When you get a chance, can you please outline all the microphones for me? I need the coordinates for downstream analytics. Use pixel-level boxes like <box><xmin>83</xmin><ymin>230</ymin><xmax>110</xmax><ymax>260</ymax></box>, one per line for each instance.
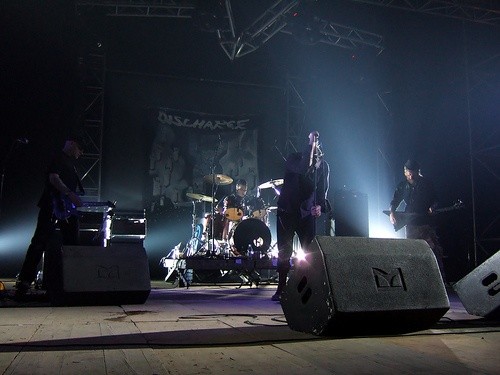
<box><xmin>316</xmin><ymin>145</ymin><xmax>324</xmax><ymax>155</ymax></box>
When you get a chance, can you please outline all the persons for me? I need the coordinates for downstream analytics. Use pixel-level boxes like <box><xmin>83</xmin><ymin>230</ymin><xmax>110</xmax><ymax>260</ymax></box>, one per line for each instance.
<box><xmin>272</xmin><ymin>132</ymin><xmax>330</xmax><ymax>300</ymax></box>
<box><xmin>17</xmin><ymin>135</ymin><xmax>88</xmax><ymax>293</ymax></box>
<box><xmin>388</xmin><ymin>160</ymin><xmax>446</xmax><ymax>285</ymax></box>
<box><xmin>215</xmin><ymin>179</ymin><xmax>254</xmax><ymax>241</ymax></box>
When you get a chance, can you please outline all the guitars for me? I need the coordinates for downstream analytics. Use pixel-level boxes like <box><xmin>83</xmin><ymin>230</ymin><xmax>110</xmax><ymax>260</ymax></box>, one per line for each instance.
<box><xmin>51</xmin><ymin>194</ymin><xmax>117</xmax><ymax>218</ymax></box>
<box><xmin>382</xmin><ymin>199</ymin><xmax>464</xmax><ymax>232</ymax></box>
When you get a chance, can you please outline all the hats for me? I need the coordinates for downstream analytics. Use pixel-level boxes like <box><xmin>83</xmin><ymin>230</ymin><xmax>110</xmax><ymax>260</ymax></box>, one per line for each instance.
<box><xmin>65</xmin><ymin>135</ymin><xmax>87</xmax><ymax>153</ymax></box>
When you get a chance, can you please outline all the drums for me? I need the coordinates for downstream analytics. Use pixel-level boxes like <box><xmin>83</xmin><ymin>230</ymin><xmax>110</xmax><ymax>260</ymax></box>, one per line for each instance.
<box><xmin>197</xmin><ymin>212</ymin><xmax>226</xmax><ymax>238</ymax></box>
<box><xmin>224</xmin><ymin>196</ymin><xmax>244</xmax><ymax>220</ymax></box>
<box><xmin>228</xmin><ymin>218</ymin><xmax>272</xmax><ymax>258</ymax></box>
<box><xmin>245</xmin><ymin>196</ymin><xmax>267</xmax><ymax>218</ymax></box>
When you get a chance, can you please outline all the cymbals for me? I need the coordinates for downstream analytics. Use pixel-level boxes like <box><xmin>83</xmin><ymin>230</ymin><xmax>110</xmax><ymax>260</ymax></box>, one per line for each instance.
<box><xmin>204</xmin><ymin>174</ymin><xmax>233</xmax><ymax>186</ymax></box>
<box><xmin>186</xmin><ymin>192</ymin><xmax>219</xmax><ymax>203</ymax></box>
<box><xmin>258</xmin><ymin>178</ymin><xmax>283</xmax><ymax>189</ymax></box>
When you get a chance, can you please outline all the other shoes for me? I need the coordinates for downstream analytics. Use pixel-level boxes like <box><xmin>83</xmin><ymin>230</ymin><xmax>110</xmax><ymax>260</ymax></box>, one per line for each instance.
<box><xmin>16</xmin><ymin>279</ymin><xmax>29</xmax><ymax>291</ymax></box>
<box><xmin>272</xmin><ymin>291</ymin><xmax>282</xmax><ymax>300</ymax></box>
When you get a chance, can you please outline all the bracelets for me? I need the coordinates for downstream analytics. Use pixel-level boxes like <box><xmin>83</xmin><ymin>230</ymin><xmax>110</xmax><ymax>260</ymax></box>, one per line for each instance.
<box><xmin>64</xmin><ymin>189</ymin><xmax>72</xmax><ymax>197</ymax></box>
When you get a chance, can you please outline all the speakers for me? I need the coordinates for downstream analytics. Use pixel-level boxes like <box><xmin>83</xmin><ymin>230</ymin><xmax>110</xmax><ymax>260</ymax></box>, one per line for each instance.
<box><xmin>279</xmin><ymin>235</ymin><xmax>451</xmax><ymax>338</ymax></box>
<box><xmin>452</xmin><ymin>250</ymin><xmax>500</xmax><ymax>327</ymax></box>
<box><xmin>45</xmin><ymin>213</ymin><xmax>152</xmax><ymax>306</ymax></box>
<box><xmin>315</xmin><ymin>192</ymin><xmax>369</xmax><ymax>237</ymax></box>
